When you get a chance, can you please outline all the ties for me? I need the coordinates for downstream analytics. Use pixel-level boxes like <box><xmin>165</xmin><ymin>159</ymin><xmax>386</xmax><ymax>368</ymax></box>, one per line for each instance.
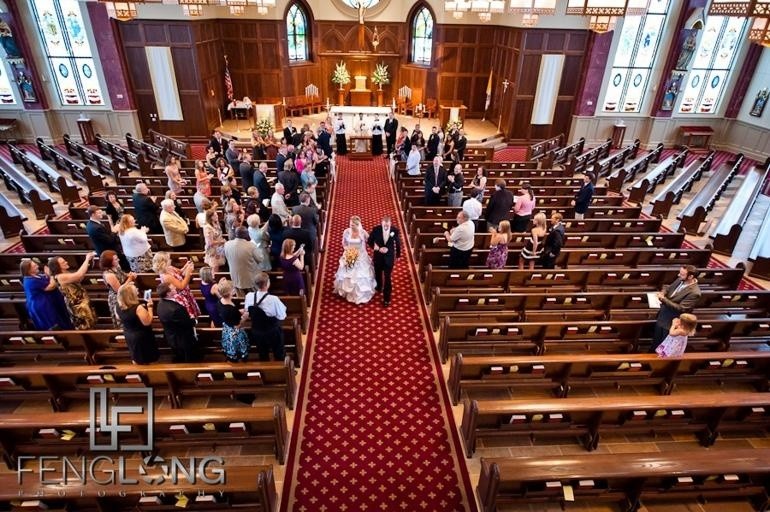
<box><xmin>435</xmin><ymin>168</ymin><xmax>438</xmax><ymax>186</ymax></box>
<box><xmin>384</xmin><ymin>231</ymin><xmax>388</xmax><ymax>245</ymax></box>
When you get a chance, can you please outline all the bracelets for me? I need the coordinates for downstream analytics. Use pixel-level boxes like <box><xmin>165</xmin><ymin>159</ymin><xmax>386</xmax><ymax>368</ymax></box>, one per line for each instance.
<box><xmin>377</xmin><ymin>247</ymin><xmax>381</xmax><ymax>252</ymax></box>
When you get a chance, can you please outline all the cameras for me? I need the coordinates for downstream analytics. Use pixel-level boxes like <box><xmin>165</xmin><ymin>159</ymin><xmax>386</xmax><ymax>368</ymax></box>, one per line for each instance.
<box><xmin>92</xmin><ymin>251</ymin><xmax>97</xmax><ymax>256</ymax></box>
<box><xmin>301</xmin><ymin>244</ymin><xmax>304</xmax><ymax>248</ymax></box>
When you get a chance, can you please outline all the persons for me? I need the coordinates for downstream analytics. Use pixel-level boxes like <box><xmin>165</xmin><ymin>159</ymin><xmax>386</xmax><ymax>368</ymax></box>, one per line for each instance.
<box><xmin>675</xmin><ymin>29</ymin><xmax>697</xmax><ymax>70</ymax></box>
<box><xmin>655</xmin><ymin>312</ymin><xmax>697</xmax><ymax>360</ymax></box>
<box><xmin>649</xmin><ymin>264</ymin><xmax>700</xmax><ymax>354</ymax></box>
<box><xmin>370</xmin><ymin>113</ymin><xmax>384</xmax><ymax>155</ymax></box>
<box><xmin>368</xmin><ymin>217</ymin><xmax>400</xmax><ymax>307</ymax></box>
<box><xmin>570</xmin><ymin>173</ymin><xmax>595</xmax><ymax>225</ymax></box>
<box><xmin>395</xmin><ymin>121</ymin><xmax>569</xmax><ymax>270</ymax></box>
<box><xmin>384</xmin><ymin>112</ymin><xmax>397</xmax><ymax>160</ymax></box>
<box><xmin>354</xmin><ymin>112</ymin><xmax>370</xmax><ymax>152</ymax></box>
<box><xmin>334</xmin><ymin>113</ymin><xmax>348</xmax><ymax>156</ymax></box>
<box><xmin>334</xmin><ymin>215</ymin><xmax>389</xmax><ymax>305</ymax></box>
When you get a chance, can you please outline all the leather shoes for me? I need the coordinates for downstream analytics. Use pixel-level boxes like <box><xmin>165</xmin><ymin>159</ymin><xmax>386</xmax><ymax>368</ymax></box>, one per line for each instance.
<box><xmin>384</xmin><ymin>300</ymin><xmax>389</xmax><ymax>307</ymax></box>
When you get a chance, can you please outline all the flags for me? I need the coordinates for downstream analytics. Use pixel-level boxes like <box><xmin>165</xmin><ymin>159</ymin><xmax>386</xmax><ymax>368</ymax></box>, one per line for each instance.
<box><xmin>224</xmin><ymin>61</ymin><xmax>234</xmax><ymax>102</ymax></box>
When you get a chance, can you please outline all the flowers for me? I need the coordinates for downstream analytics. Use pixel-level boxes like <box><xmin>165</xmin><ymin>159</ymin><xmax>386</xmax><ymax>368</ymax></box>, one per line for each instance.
<box><xmin>253</xmin><ymin>112</ymin><xmax>274</xmax><ymax>138</ymax></box>
<box><xmin>341</xmin><ymin>246</ymin><xmax>360</xmax><ymax>271</ymax></box>
<box><xmin>443</xmin><ymin>117</ymin><xmax>464</xmax><ymax>134</ymax></box>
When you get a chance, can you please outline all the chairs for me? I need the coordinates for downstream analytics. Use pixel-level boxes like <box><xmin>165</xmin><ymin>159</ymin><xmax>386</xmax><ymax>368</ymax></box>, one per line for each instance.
<box><xmin>391</xmin><ymin>95</ymin><xmax>463</xmax><ymax>122</ymax></box>
<box><xmin>257</xmin><ymin>94</ymin><xmax>324</xmax><ymax>116</ymax></box>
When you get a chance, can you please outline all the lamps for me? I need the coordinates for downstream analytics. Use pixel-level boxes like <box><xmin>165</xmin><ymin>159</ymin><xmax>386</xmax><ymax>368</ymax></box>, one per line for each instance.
<box><xmin>564</xmin><ymin>0</ymin><xmax>652</xmax><ymax>36</ymax></box>
<box><xmin>93</xmin><ymin>0</ymin><xmax>162</xmax><ymax>25</ymax></box>
<box><xmin>445</xmin><ymin>0</ymin><xmax>558</xmax><ymax>29</ymax></box>
<box><xmin>160</xmin><ymin>0</ymin><xmax>221</xmax><ymax>20</ymax></box>
<box><xmin>216</xmin><ymin>1</ymin><xmax>256</xmax><ymax>17</ymax></box>
<box><xmin>247</xmin><ymin>0</ymin><xmax>278</xmax><ymax>16</ymax></box>
<box><xmin>707</xmin><ymin>1</ymin><xmax>770</xmax><ymax>52</ymax></box>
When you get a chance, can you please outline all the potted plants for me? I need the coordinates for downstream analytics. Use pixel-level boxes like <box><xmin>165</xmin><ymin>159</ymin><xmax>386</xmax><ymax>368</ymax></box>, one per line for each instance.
<box><xmin>366</xmin><ymin>61</ymin><xmax>392</xmax><ymax>91</ymax></box>
<box><xmin>331</xmin><ymin>59</ymin><xmax>351</xmax><ymax>89</ymax></box>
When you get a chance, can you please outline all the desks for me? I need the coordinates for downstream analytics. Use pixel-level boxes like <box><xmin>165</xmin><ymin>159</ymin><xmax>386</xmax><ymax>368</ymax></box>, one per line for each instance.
<box><xmin>348</xmin><ymin>136</ymin><xmax>375</xmax><ymax>162</ymax></box>
<box><xmin>227</xmin><ymin>98</ymin><xmax>256</xmax><ymax>120</ymax></box>
<box><xmin>330</xmin><ymin>106</ymin><xmax>393</xmax><ymax>131</ymax></box>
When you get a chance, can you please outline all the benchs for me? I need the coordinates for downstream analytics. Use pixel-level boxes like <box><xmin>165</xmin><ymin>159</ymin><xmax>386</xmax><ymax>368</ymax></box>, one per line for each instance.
<box><xmin>390</xmin><ymin>132</ymin><xmax>769</xmax><ymax>508</ymax></box>
<box><xmin>0</xmin><ymin>125</ymin><xmax>341</xmax><ymax>511</ymax></box>
